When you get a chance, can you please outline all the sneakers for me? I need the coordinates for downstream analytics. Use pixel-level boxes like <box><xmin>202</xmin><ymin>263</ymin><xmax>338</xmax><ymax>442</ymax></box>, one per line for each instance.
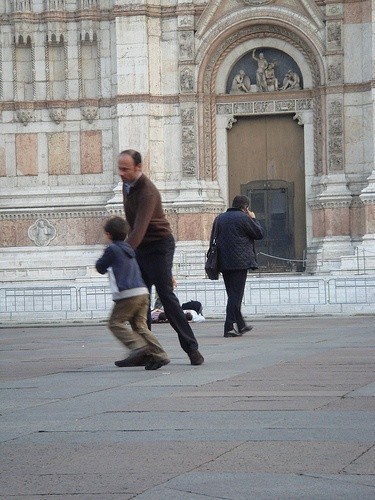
<box><xmin>115</xmin><ymin>358</ymin><xmax>146</xmax><ymax>366</ymax></box>
<box><xmin>188</xmin><ymin>350</ymin><xmax>204</xmax><ymax>364</ymax></box>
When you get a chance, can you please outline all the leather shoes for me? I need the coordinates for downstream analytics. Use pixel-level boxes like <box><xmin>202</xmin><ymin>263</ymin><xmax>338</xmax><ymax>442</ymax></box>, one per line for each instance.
<box><xmin>240</xmin><ymin>325</ymin><xmax>253</xmax><ymax>334</ymax></box>
<box><xmin>225</xmin><ymin>328</ymin><xmax>241</xmax><ymax>337</ymax></box>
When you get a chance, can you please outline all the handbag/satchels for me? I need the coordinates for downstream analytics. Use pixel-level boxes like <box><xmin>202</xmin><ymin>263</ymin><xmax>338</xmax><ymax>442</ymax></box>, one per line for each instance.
<box><xmin>205</xmin><ymin>243</ymin><xmax>219</xmax><ymax>280</ymax></box>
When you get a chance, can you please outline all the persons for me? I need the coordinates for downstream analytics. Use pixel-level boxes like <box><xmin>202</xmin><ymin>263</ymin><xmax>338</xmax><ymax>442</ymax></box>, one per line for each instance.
<box><xmin>209</xmin><ymin>194</ymin><xmax>265</xmax><ymax>337</ymax></box>
<box><xmin>118</xmin><ymin>149</ymin><xmax>205</xmax><ymax>365</ymax></box>
<box><xmin>155</xmin><ymin>278</ymin><xmax>177</xmax><ymax>310</ymax></box>
<box><xmin>231</xmin><ymin>48</ymin><xmax>300</xmax><ymax>93</ymax></box>
<box><xmin>95</xmin><ymin>217</ymin><xmax>171</xmax><ymax>371</ymax></box>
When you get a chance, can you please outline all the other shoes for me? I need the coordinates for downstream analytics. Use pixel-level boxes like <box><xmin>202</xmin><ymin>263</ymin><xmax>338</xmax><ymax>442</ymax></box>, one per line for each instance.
<box><xmin>127</xmin><ymin>346</ymin><xmax>154</xmax><ymax>363</ymax></box>
<box><xmin>144</xmin><ymin>358</ymin><xmax>170</xmax><ymax>370</ymax></box>
